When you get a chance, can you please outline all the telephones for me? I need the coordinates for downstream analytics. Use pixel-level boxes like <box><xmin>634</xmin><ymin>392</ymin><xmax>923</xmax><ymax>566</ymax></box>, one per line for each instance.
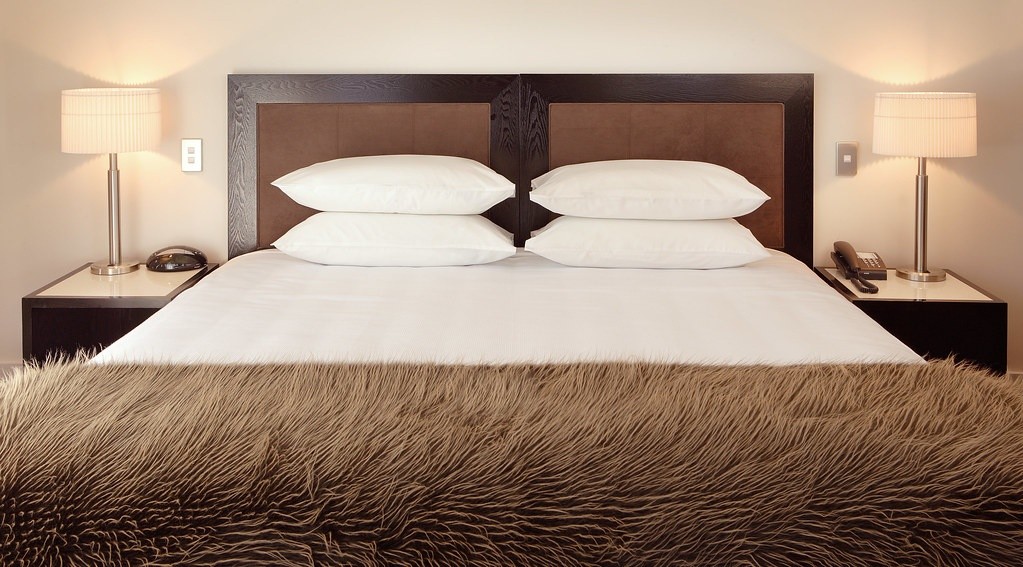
<box><xmin>831</xmin><ymin>240</ymin><xmax>887</xmax><ymax>280</ymax></box>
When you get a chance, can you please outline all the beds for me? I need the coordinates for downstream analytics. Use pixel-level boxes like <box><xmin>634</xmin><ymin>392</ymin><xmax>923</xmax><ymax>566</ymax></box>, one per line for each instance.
<box><xmin>2</xmin><ymin>72</ymin><xmax>1022</xmax><ymax>565</ymax></box>
